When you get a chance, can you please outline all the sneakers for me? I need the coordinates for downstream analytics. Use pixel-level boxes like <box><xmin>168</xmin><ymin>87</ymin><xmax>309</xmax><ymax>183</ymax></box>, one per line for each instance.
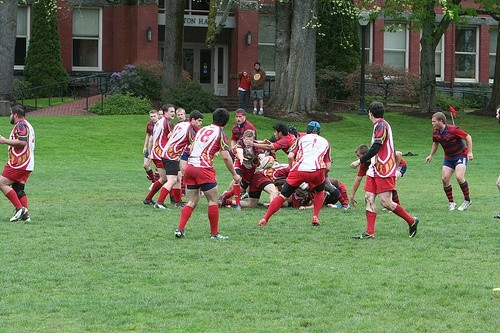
<box><xmin>10</xmin><ymin>206</ymin><xmax>28</xmax><ymax>221</ymax></box>
<box><xmin>21</xmin><ymin>216</ymin><xmax>30</xmax><ymax>222</ymax></box>
<box><xmin>409</xmin><ymin>217</ymin><xmax>419</xmax><ymax>238</ymax></box>
<box><xmin>458</xmin><ymin>200</ymin><xmax>472</xmax><ymax>211</ymax></box>
<box><xmin>449</xmin><ymin>203</ymin><xmax>456</xmax><ymax>211</ymax></box>
<box><xmin>352</xmin><ymin>232</ymin><xmax>375</xmax><ymax>239</ymax></box>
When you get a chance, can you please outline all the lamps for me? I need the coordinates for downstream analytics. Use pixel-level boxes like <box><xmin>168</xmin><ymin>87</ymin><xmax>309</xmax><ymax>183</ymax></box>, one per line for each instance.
<box><xmin>246</xmin><ymin>31</ymin><xmax>252</xmax><ymax>45</ymax></box>
<box><xmin>147</xmin><ymin>26</ymin><xmax>152</xmax><ymax>42</ymax></box>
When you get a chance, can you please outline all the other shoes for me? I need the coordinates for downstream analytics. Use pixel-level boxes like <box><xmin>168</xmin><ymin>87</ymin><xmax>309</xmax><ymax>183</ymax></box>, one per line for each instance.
<box><xmin>258</xmin><ymin>219</ymin><xmax>267</xmax><ymax>226</ymax></box>
<box><xmin>253</xmin><ymin>111</ymin><xmax>258</xmax><ymax>114</ymax></box>
<box><xmin>143</xmin><ymin>201</ymin><xmax>155</xmax><ymax>204</ymax></box>
<box><xmin>342</xmin><ymin>204</ymin><xmax>349</xmax><ymax>210</ymax></box>
<box><xmin>209</xmin><ymin>233</ymin><xmax>225</xmax><ymax>240</ymax></box>
<box><xmin>174</xmin><ymin>202</ymin><xmax>187</xmax><ymax>207</ymax></box>
<box><xmin>258</xmin><ymin>110</ymin><xmax>263</xmax><ymax>114</ymax></box>
<box><xmin>312</xmin><ymin>216</ymin><xmax>321</xmax><ymax>225</ymax></box>
<box><xmin>175</xmin><ymin>228</ymin><xmax>184</xmax><ymax>238</ymax></box>
<box><xmin>219</xmin><ymin>190</ymin><xmax>247</xmax><ymax>211</ymax></box>
<box><xmin>154</xmin><ymin>203</ymin><xmax>166</xmax><ymax>208</ymax></box>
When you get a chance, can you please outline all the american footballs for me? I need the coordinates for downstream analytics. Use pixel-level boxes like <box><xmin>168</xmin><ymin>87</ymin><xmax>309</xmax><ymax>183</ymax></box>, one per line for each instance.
<box><xmin>261</xmin><ymin>156</ymin><xmax>274</xmax><ymax>169</ymax></box>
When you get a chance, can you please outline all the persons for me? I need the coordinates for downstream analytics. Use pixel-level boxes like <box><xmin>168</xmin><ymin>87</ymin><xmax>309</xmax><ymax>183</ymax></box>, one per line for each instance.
<box><xmin>0</xmin><ymin>105</ymin><xmax>35</xmax><ymax>223</ymax></box>
<box><xmin>426</xmin><ymin>112</ymin><xmax>474</xmax><ymax>212</ymax></box>
<box><xmin>494</xmin><ymin>107</ymin><xmax>500</xmax><ymax>219</ymax></box>
<box><xmin>142</xmin><ymin>62</ymin><xmax>419</xmax><ymax>240</ymax></box>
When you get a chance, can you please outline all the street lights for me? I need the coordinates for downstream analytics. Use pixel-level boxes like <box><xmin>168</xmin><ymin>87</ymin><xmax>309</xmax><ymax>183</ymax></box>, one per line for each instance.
<box><xmin>356</xmin><ymin>7</ymin><xmax>372</xmax><ymax>115</ymax></box>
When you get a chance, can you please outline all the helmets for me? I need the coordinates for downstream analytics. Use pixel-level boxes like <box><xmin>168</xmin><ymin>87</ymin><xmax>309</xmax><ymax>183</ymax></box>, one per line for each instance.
<box><xmin>307</xmin><ymin>121</ymin><xmax>320</xmax><ymax>135</ymax></box>
